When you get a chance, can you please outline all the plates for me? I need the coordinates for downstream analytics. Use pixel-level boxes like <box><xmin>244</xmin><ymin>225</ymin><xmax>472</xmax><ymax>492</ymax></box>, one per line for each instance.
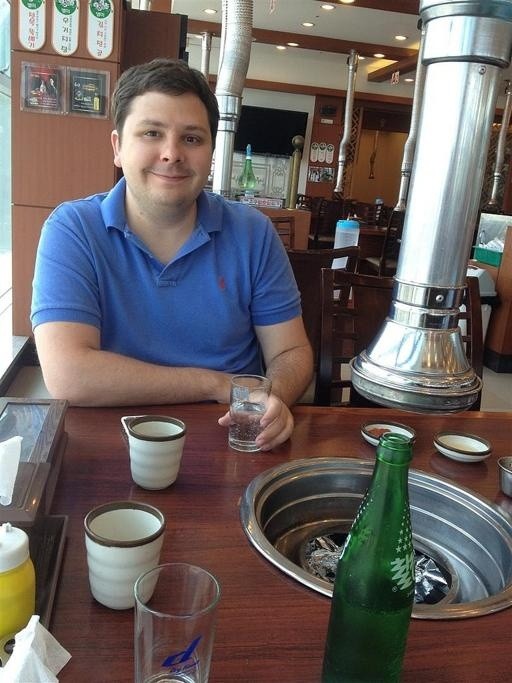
<box><xmin>361</xmin><ymin>420</ymin><xmax>417</xmax><ymax>449</ymax></box>
<box><xmin>432</xmin><ymin>429</ymin><xmax>492</xmax><ymax>463</ymax></box>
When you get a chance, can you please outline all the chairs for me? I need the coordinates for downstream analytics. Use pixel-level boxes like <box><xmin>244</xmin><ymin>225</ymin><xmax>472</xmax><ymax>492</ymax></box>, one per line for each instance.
<box><xmin>249</xmin><ymin>190</ymin><xmax>490</xmax><ymax>410</ymax></box>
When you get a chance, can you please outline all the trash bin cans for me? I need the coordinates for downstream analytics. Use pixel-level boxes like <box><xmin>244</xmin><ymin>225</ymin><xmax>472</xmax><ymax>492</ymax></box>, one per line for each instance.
<box><xmin>457</xmin><ymin>264</ymin><xmax>498</xmax><ymax>355</ymax></box>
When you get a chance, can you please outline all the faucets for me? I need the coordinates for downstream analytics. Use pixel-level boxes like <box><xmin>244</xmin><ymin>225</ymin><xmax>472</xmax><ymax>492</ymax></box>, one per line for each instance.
<box><xmin>477</xmin><ymin>228</ymin><xmax>486</xmax><ymax>243</ymax></box>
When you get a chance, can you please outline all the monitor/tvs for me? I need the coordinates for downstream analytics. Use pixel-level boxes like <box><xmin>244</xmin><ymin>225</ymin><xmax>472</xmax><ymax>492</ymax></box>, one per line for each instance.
<box><xmin>234</xmin><ymin>102</ymin><xmax>309</xmax><ymax>159</ymax></box>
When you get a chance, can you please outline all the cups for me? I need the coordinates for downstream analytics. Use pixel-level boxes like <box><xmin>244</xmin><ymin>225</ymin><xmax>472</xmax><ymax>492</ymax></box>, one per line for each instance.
<box><xmin>228</xmin><ymin>374</ymin><xmax>273</xmax><ymax>452</ymax></box>
<box><xmin>495</xmin><ymin>455</ymin><xmax>512</xmax><ymax>500</ymax></box>
<box><xmin>120</xmin><ymin>412</ymin><xmax>188</xmax><ymax>490</ymax></box>
<box><xmin>133</xmin><ymin>563</ymin><xmax>220</xmax><ymax>682</ymax></box>
<box><xmin>82</xmin><ymin>500</ymin><xmax>166</xmax><ymax>610</ymax></box>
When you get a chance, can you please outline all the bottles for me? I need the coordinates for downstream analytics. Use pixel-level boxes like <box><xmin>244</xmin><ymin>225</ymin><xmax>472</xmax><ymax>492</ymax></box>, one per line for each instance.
<box><xmin>322</xmin><ymin>433</ymin><xmax>418</xmax><ymax>682</ymax></box>
<box><xmin>238</xmin><ymin>142</ymin><xmax>256</xmax><ymax>189</ymax></box>
<box><xmin>0</xmin><ymin>519</ymin><xmax>38</xmax><ymax>670</ymax></box>
<box><xmin>329</xmin><ymin>220</ymin><xmax>361</xmax><ymax>299</ymax></box>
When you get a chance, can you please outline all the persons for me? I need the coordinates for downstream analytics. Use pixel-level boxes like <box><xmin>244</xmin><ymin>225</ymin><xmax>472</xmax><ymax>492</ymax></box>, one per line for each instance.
<box><xmin>30</xmin><ymin>58</ymin><xmax>315</xmax><ymax>452</ymax></box>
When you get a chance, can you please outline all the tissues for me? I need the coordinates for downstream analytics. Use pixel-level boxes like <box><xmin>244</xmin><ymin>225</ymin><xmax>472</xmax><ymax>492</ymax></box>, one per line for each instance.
<box><xmin>0</xmin><ymin>430</ymin><xmax>52</xmax><ymax>547</ymax></box>
<box><xmin>0</xmin><ymin>620</ymin><xmax>66</xmax><ymax>683</ymax></box>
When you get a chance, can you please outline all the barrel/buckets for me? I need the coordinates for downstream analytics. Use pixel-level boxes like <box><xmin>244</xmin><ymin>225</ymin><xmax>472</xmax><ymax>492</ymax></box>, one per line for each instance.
<box><xmin>330</xmin><ymin>215</ymin><xmax>361</xmax><ymax>271</ymax></box>
